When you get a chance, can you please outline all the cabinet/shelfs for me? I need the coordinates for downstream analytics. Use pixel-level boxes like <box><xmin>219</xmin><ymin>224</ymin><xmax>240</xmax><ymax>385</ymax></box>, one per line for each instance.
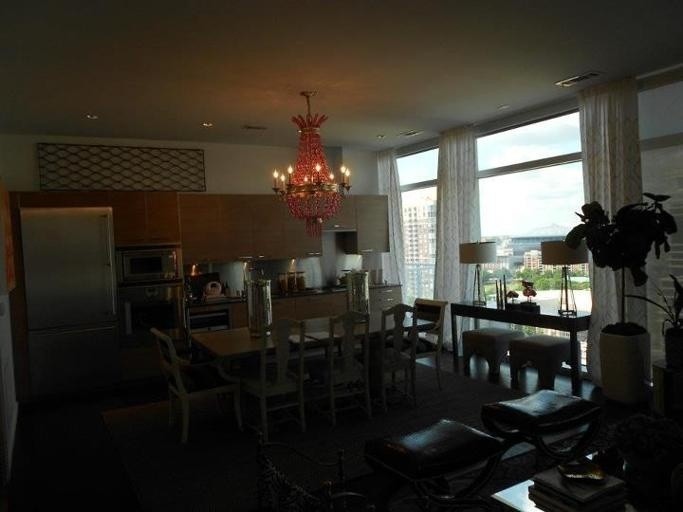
<box><xmin>110</xmin><ymin>191</ymin><xmax>182</xmax><ymax>248</ymax></box>
<box><xmin>176</xmin><ymin>194</ymin><xmax>393</xmax><ymax>262</ymax></box>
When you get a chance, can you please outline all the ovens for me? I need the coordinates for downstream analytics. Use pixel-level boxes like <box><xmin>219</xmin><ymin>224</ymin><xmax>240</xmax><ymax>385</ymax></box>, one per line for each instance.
<box><xmin>118</xmin><ymin>286</ymin><xmax>185</xmax><ymax>337</ymax></box>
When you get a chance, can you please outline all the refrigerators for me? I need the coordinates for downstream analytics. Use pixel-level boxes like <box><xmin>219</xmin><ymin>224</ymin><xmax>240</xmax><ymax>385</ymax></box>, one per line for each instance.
<box><xmin>17</xmin><ymin>206</ymin><xmax>117</xmax><ymax>414</ymax></box>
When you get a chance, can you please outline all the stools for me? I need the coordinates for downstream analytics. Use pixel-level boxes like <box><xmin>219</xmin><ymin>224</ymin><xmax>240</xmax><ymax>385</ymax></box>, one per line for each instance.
<box><xmin>370</xmin><ymin>415</ymin><xmax>510</xmax><ymax>511</ymax></box>
<box><xmin>460</xmin><ymin>326</ymin><xmax>523</xmax><ymax>384</ymax></box>
<box><xmin>477</xmin><ymin>383</ymin><xmax>601</xmax><ymax>472</ymax></box>
<box><xmin>508</xmin><ymin>335</ymin><xmax>581</xmax><ymax>391</ymax></box>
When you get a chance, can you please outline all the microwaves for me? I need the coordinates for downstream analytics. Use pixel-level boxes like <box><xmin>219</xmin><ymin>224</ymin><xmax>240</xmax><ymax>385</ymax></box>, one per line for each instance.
<box><xmin>122</xmin><ymin>248</ymin><xmax>177</xmax><ymax>278</ymax></box>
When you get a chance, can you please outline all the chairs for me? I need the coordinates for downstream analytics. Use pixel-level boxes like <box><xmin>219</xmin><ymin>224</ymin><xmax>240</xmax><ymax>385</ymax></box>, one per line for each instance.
<box><xmin>245</xmin><ymin>318</ymin><xmax>310</xmax><ymax>438</ymax></box>
<box><xmin>379</xmin><ymin>303</ymin><xmax>420</xmax><ymax>385</ymax></box>
<box><xmin>310</xmin><ymin>311</ymin><xmax>370</xmax><ymax>426</ymax></box>
<box><xmin>370</xmin><ymin>337</ymin><xmax>416</xmax><ymax>415</ymax></box>
<box><xmin>252</xmin><ymin>430</ymin><xmax>377</xmax><ymax>512</ymax></box>
<box><xmin>409</xmin><ymin>302</ymin><xmax>444</xmax><ymax>390</ymax></box>
<box><xmin>150</xmin><ymin>326</ymin><xmax>246</xmax><ymax>440</ymax></box>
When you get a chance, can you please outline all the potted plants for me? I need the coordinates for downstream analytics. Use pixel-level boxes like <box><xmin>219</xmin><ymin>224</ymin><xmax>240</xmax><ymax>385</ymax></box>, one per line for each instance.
<box><xmin>619</xmin><ymin>271</ymin><xmax>682</xmax><ymax>371</ymax></box>
<box><xmin>569</xmin><ymin>186</ymin><xmax>677</xmax><ymax>408</ymax></box>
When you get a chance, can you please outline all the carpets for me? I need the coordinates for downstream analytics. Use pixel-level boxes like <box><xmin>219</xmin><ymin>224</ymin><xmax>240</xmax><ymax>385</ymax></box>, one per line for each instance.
<box><xmin>385</xmin><ymin>427</ymin><xmax>615</xmax><ymax>512</ymax></box>
<box><xmin>102</xmin><ymin>354</ymin><xmax>527</xmax><ymax>510</ymax></box>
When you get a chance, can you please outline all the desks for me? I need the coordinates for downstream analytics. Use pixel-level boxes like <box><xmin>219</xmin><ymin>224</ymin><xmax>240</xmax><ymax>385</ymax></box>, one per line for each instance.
<box><xmin>489</xmin><ymin>446</ymin><xmax>670</xmax><ymax>512</ymax></box>
<box><xmin>449</xmin><ymin>299</ymin><xmax>593</xmax><ymax>398</ymax></box>
<box><xmin>192</xmin><ymin>312</ymin><xmax>437</xmax><ymax>387</ymax></box>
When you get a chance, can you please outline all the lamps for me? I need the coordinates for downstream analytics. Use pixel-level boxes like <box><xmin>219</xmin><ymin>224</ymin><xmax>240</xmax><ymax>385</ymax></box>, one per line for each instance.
<box><xmin>459</xmin><ymin>239</ymin><xmax>498</xmax><ymax>305</ymax></box>
<box><xmin>537</xmin><ymin>238</ymin><xmax>587</xmax><ymax>316</ymax></box>
<box><xmin>266</xmin><ymin>87</ymin><xmax>354</xmax><ymax>240</ymax></box>
